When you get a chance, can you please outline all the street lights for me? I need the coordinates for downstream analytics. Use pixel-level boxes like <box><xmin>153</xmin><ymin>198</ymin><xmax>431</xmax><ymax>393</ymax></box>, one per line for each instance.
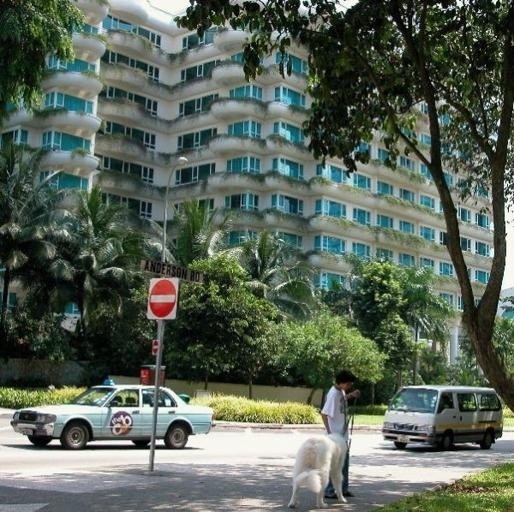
<box><xmin>162</xmin><ymin>155</ymin><xmax>190</xmax><ymax>263</ymax></box>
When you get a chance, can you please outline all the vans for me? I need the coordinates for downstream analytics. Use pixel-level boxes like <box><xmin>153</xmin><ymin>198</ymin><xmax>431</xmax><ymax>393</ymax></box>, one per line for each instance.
<box><xmin>381</xmin><ymin>384</ymin><xmax>505</xmax><ymax>450</ymax></box>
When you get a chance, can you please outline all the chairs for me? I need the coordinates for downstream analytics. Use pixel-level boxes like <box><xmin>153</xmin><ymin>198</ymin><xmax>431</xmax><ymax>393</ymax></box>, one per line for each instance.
<box><xmin>124</xmin><ymin>397</ymin><xmax>136</xmax><ymax>406</ymax></box>
<box><xmin>113</xmin><ymin>396</ymin><xmax>122</xmax><ymax>403</ymax></box>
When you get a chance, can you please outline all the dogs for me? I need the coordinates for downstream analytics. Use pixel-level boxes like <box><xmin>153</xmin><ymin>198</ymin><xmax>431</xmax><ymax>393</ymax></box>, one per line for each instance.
<box><xmin>287</xmin><ymin>432</ymin><xmax>347</xmax><ymax>508</ymax></box>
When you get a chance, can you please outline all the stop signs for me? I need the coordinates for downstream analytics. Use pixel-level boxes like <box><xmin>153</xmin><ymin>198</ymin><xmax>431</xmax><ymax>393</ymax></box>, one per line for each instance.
<box><xmin>146</xmin><ymin>277</ymin><xmax>181</xmax><ymax>322</ymax></box>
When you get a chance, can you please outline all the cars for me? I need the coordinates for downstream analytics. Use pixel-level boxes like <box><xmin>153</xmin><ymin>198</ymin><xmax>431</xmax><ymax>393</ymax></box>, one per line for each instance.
<box><xmin>9</xmin><ymin>383</ymin><xmax>217</xmax><ymax>450</ymax></box>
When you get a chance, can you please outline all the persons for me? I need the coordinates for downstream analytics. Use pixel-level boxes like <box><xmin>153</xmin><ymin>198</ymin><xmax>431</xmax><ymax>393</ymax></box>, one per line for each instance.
<box><xmin>320</xmin><ymin>370</ymin><xmax>361</xmax><ymax>499</ymax></box>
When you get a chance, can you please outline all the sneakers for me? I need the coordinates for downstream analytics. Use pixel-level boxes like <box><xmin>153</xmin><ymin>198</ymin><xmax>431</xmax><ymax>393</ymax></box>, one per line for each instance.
<box><xmin>325</xmin><ymin>492</ymin><xmax>354</xmax><ymax>498</ymax></box>
